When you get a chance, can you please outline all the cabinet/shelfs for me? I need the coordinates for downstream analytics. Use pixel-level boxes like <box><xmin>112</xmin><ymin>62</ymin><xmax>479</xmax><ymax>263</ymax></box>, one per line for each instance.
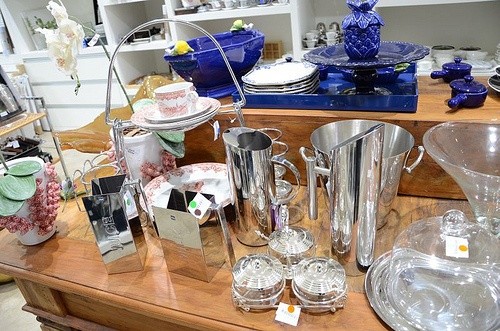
<box><xmin>0</xmin><ymin>0</ymin><xmax>317</xmax><ymax>133</ymax></box>
<box><xmin>0</xmin><ymin>96</ymin><xmax>70</xmax><ymax>178</ymax></box>
<box><xmin>1</xmin><ymin>73</ymin><xmax>500</xmax><ymax>331</ymax></box>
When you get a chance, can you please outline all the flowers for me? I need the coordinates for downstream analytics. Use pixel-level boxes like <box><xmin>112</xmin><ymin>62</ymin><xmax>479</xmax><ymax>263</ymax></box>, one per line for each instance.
<box><xmin>172</xmin><ymin>37</ymin><xmax>194</xmax><ymax>57</ymax></box>
<box><xmin>228</xmin><ymin>16</ymin><xmax>244</xmax><ymax>31</ymax></box>
<box><xmin>24</xmin><ymin>0</ymin><xmax>186</xmax><ymax>159</ymax></box>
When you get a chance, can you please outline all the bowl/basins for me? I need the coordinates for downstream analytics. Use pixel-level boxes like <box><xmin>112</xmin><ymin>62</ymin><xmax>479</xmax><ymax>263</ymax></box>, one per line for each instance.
<box><xmin>162</xmin><ymin>30</ymin><xmax>265</xmax><ymax>98</ymax></box>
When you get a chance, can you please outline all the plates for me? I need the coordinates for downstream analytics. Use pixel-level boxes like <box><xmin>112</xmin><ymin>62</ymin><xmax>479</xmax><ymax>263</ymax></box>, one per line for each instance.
<box><xmin>303</xmin><ymin>40</ymin><xmax>430</xmax><ymax>68</ymax></box>
<box><xmin>487</xmin><ymin>74</ymin><xmax>500</xmax><ymax>92</ymax></box>
<box><xmin>241</xmin><ymin>58</ymin><xmax>320</xmax><ymax>95</ymax></box>
<box><xmin>130</xmin><ymin>96</ymin><xmax>221</xmax><ymax>131</ymax></box>
<box><xmin>139</xmin><ymin>162</ymin><xmax>232</xmax><ymax>220</ymax></box>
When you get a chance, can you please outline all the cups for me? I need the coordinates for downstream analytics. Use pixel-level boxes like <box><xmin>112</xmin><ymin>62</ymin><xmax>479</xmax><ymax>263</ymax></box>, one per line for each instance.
<box><xmin>155</xmin><ymin>82</ymin><xmax>199</xmax><ymax>119</ymax></box>
<box><xmin>305</xmin><ymin>32</ymin><xmax>318</xmax><ymax>48</ymax></box>
<box><xmin>341</xmin><ymin>0</ymin><xmax>385</xmax><ymax>60</ymax></box>
<box><xmin>222</xmin><ymin>126</ymin><xmax>278</xmax><ymax>246</ymax></box>
<box><xmin>109</xmin><ymin>121</ymin><xmax>163</xmax><ymax>189</ymax></box>
<box><xmin>330</xmin><ymin>123</ymin><xmax>384</xmax><ymax>277</ymax></box>
<box><xmin>300</xmin><ymin>119</ymin><xmax>425</xmax><ymax>231</ymax></box>
<box><xmin>432</xmin><ymin>45</ymin><xmax>455</xmax><ymax>57</ymax></box>
<box><xmin>460</xmin><ymin>47</ymin><xmax>481</xmax><ymax>57</ymax></box>
<box><xmin>326</xmin><ymin>32</ymin><xmax>338</xmax><ymax>46</ymax></box>
<box><xmin>0</xmin><ymin>156</ymin><xmax>56</xmax><ymax>246</ymax></box>
<box><xmin>422</xmin><ymin>119</ymin><xmax>500</xmax><ymax>237</ymax></box>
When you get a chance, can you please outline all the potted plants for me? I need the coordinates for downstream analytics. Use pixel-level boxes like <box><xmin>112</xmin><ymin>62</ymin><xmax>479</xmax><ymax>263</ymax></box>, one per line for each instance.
<box><xmin>1</xmin><ymin>153</ymin><xmax>59</xmax><ymax>246</ymax></box>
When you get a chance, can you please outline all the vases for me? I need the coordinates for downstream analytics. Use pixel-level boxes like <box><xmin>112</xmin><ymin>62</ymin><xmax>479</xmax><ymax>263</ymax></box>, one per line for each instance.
<box><xmin>107</xmin><ymin>123</ymin><xmax>177</xmax><ymax>194</ymax></box>
